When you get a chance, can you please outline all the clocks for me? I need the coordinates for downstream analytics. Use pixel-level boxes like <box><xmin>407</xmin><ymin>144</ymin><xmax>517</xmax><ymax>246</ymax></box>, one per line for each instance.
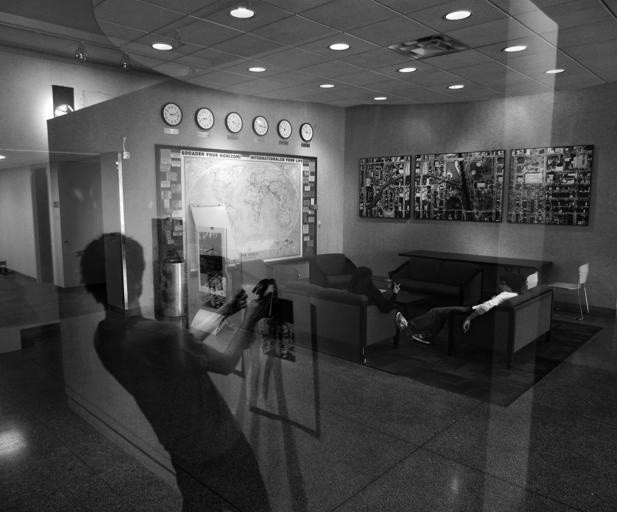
<box><xmin>253</xmin><ymin>115</ymin><xmax>270</xmax><ymax>136</ymax></box>
<box><xmin>226</xmin><ymin>111</ymin><xmax>244</xmax><ymax>133</ymax></box>
<box><xmin>277</xmin><ymin>120</ymin><xmax>293</xmax><ymax>138</ymax></box>
<box><xmin>195</xmin><ymin>107</ymin><xmax>216</xmax><ymax>130</ymax></box>
<box><xmin>299</xmin><ymin>123</ymin><xmax>314</xmax><ymax>142</ymax></box>
<box><xmin>161</xmin><ymin>102</ymin><xmax>184</xmax><ymax>127</ymax></box>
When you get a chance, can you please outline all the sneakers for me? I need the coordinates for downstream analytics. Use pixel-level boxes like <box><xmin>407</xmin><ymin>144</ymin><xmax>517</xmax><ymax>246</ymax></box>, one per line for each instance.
<box><xmin>396</xmin><ymin>312</ymin><xmax>412</xmax><ymax>337</ymax></box>
<box><xmin>408</xmin><ymin>332</ymin><xmax>432</xmax><ymax>345</ymax></box>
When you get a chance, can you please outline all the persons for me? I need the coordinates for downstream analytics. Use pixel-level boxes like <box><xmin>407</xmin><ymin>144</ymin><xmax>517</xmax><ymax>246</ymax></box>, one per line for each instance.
<box><xmin>395</xmin><ymin>270</ymin><xmax>523</xmax><ymax>345</ymax></box>
<box><xmin>75</xmin><ymin>231</ymin><xmax>271</xmax><ymax>511</ymax></box>
<box><xmin>347</xmin><ymin>265</ymin><xmax>400</xmax><ymax>314</ymax></box>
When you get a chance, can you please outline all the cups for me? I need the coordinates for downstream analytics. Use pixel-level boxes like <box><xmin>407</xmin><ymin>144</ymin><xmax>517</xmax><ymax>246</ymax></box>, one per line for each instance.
<box><xmin>384</xmin><ymin>278</ymin><xmax>392</xmax><ymax>290</ymax></box>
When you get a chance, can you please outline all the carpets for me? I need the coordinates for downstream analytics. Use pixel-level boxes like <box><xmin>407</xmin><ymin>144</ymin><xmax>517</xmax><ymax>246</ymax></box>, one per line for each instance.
<box><xmin>292</xmin><ymin>319</ymin><xmax>603</xmax><ymax>408</ymax></box>
